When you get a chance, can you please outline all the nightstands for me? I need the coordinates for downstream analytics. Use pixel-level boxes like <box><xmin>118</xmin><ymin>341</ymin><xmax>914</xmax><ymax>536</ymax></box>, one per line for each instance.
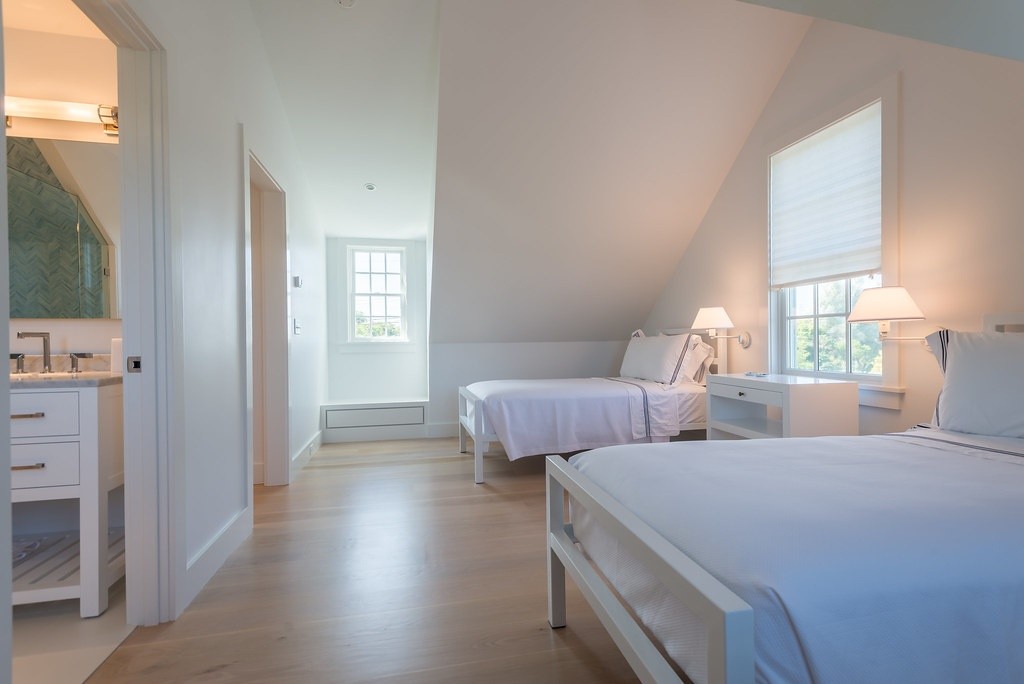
<box><xmin>705</xmin><ymin>373</ymin><xmax>860</xmax><ymax>440</ymax></box>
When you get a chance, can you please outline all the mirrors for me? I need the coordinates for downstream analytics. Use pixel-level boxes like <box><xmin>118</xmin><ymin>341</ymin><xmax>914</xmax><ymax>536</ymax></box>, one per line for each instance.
<box><xmin>5</xmin><ymin>134</ymin><xmax>121</xmax><ymax>320</ymax></box>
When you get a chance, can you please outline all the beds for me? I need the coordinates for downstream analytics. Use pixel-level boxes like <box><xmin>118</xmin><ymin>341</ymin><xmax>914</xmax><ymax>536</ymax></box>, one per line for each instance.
<box><xmin>547</xmin><ymin>324</ymin><xmax>1024</xmax><ymax>684</ymax></box>
<box><xmin>458</xmin><ymin>328</ymin><xmax>728</xmax><ymax>484</ymax></box>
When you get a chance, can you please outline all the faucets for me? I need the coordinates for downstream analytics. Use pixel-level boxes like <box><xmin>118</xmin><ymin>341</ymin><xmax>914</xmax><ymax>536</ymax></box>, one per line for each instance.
<box><xmin>17</xmin><ymin>331</ymin><xmax>54</xmax><ymax>373</ymax></box>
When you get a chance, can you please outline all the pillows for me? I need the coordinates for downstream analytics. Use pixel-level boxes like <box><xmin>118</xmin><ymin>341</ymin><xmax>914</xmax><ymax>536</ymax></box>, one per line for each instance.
<box><xmin>658</xmin><ymin>332</ymin><xmax>715</xmax><ymax>386</ymax></box>
<box><xmin>925</xmin><ymin>329</ymin><xmax>1024</xmax><ymax>439</ymax></box>
<box><xmin>618</xmin><ymin>328</ymin><xmax>695</xmax><ymax>389</ymax></box>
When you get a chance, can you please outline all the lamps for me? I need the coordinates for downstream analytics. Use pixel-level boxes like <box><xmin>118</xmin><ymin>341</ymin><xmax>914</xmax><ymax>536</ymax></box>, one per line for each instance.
<box><xmin>691</xmin><ymin>307</ymin><xmax>752</xmax><ymax>349</ymax></box>
<box><xmin>5</xmin><ymin>95</ymin><xmax>119</xmax><ymax>135</ymax></box>
<box><xmin>846</xmin><ymin>284</ymin><xmax>926</xmax><ymax>341</ymax></box>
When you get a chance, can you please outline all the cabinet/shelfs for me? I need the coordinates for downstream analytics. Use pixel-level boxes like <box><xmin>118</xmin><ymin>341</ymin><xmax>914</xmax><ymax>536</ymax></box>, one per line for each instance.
<box><xmin>10</xmin><ymin>372</ymin><xmax>124</xmax><ymax>615</ymax></box>
